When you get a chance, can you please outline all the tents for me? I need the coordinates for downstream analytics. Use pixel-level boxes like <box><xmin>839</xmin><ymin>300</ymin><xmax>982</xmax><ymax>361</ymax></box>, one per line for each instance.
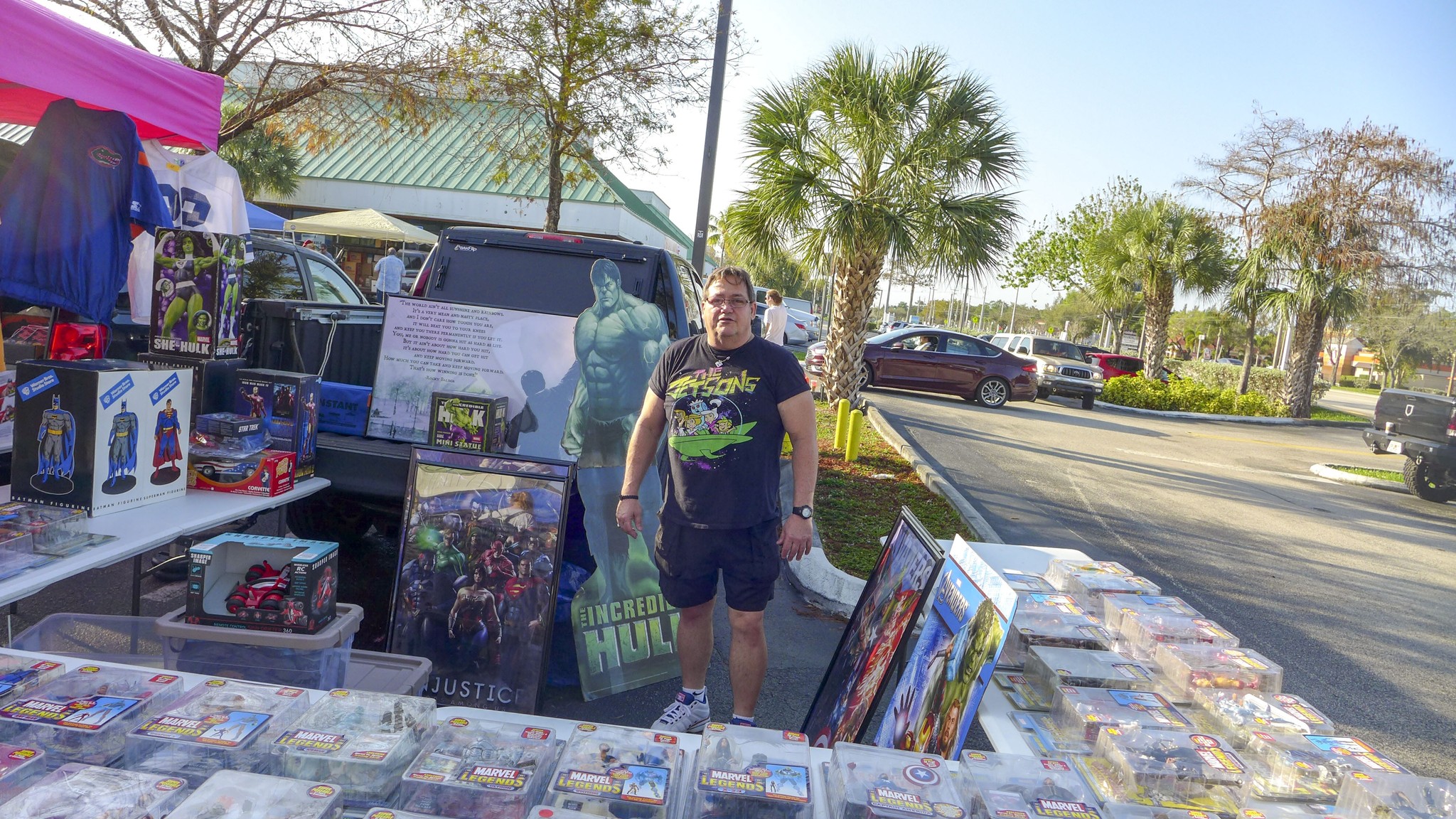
<box><xmin>281</xmin><ymin>208</ymin><xmax>438</xmax><ymax>304</ymax></box>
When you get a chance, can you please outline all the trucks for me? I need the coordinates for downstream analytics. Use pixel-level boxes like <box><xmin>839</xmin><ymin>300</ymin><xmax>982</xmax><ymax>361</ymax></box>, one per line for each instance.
<box><xmin>332</xmin><ymin>242</ymin><xmax>430</xmax><ymax>301</ymax></box>
<box><xmin>703</xmin><ymin>278</ymin><xmax>820</xmax><ymax>341</ymax></box>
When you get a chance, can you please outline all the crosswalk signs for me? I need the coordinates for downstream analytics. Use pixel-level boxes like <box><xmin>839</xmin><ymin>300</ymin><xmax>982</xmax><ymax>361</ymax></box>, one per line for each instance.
<box><xmin>1048</xmin><ymin>327</ymin><xmax>1053</xmax><ymax>332</ymax></box>
<box><xmin>973</xmin><ymin>317</ymin><xmax>978</xmax><ymax>321</ymax></box>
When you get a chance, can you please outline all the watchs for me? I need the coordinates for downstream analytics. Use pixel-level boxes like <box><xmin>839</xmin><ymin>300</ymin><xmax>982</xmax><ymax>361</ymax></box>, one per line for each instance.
<box><xmin>792</xmin><ymin>505</ymin><xmax>814</xmax><ymax>519</ymax></box>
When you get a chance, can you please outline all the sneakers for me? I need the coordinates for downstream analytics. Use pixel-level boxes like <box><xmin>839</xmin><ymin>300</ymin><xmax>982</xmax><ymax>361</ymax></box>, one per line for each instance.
<box><xmin>649</xmin><ymin>691</ymin><xmax>713</xmax><ymax>739</ymax></box>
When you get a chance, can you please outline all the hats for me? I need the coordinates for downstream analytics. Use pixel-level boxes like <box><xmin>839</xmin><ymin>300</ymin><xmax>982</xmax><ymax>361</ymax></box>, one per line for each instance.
<box><xmin>302</xmin><ymin>240</ymin><xmax>313</xmax><ymax>247</ymax></box>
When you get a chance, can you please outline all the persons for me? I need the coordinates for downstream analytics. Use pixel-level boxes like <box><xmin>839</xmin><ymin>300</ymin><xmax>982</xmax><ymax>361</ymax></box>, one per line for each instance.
<box><xmin>302</xmin><ymin>239</ymin><xmax>334</xmax><ymax>261</ymax></box>
<box><xmin>614</xmin><ymin>268</ymin><xmax>819</xmax><ymax>735</ymax></box>
<box><xmin>912</xmin><ymin>336</ymin><xmax>934</xmax><ymax>354</ymax></box>
<box><xmin>373</xmin><ymin>248</ymin><xmax>405</xmax><ymax>307</ymax></box>
<box><xmin>761</xmin><ymin>289</ymin><xmax>787</xmax><ymax>348</ymax></box>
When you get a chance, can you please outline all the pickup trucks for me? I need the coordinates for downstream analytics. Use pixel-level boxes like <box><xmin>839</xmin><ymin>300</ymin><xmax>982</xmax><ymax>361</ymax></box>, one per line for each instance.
<box><xmin>1363</xmin><ymin>387</ymin><xmax>1456</xmax><ymax>503</ymax></box>
<box><xmin>989</xmin><ymin>333</ymin><xmax>1104</xmax><ymax>410</ymax></box>
<box><xmin>196</xmin><ymin>225</ymin><xmax>766</xmax><ymax>544</ymax></box>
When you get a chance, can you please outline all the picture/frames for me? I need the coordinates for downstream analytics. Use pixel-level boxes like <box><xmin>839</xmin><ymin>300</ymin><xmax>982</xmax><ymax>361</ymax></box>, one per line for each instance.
<box><xmin>797</xmin><ymin>505</ymin><xmax>943</xmax><ymax>741</ymax></box>
<box><xmin>385</xmin><ymin>444</ymin><xmax>575</xmax><ymax>715</ymax></box>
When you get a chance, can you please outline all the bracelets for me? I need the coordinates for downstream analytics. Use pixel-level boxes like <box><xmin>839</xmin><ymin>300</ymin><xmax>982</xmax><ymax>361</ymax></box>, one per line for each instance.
<box><xmin>618</xmin><ymin>494</ymin><xmax>639</xmax><ymax>501</ymax></box>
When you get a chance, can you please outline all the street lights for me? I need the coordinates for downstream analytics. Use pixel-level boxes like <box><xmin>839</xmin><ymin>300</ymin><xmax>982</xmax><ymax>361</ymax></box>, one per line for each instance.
<box><xmin>956</xmin><ymin>274</ymin><xmax>969</xmax><ymax>332</ymax></box>
<box><xmin>1097</xmin><ymin>315</ymin><xmax>1106</xmax><ymax>347</ymax></box>
<box><xmin>876</xmin><ymin>289</ymin><xmax>883</xmax><ymax>324</ymax></box>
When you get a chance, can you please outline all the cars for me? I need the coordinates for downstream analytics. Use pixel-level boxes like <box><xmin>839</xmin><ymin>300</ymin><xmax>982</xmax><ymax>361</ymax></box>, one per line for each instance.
<box><xmin>1216</xmin><ymin>358</ymin><xmax>1244</xmax><ymax>365</ymax></box>
<box><xmin>1056</xmin><ymin>344</ymin><xmax>1113</xmax><ymax>359</ymax></box>
<box><xmin>755</xmin><ymin>301</ymin><xmax>809</xmax><ymax>345</ymax></box>
<box><xmin>887</xmin><ymin>320</ymin><xmax>913</xmax><ymax>333</ymax></box>
<box><xmin>804</xmin><ymin>328</ymin><xmax>1039</xmax><ymax>409</ymax></box>
<box><xmin>902</xmin><ymin>323</ymin><xmax>994</xmax><ymax>345</ymax></box>
<box><xmin>74</xmin><ymin>235</ymin><xmax>383</xmax><ymax>363</ymax></box>
<box><xmin>878</xmin><ymin>322</ymin><xmax>892</xmax><ymax>334</ymax></box>
<box><xmin>194</xmin><ymin>459</ymin><xmax>259</xmax><ymax>477</ymax></box>
<box><xmin>1085</xmin><ymin>353</ymin><xmax>1173</xmax><ymax>385</ymax></box>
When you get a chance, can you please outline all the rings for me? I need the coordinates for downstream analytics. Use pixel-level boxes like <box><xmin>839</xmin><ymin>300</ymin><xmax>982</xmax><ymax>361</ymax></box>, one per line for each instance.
<box><xmin>617</xmin><ymin>524</ymin><xmax>621</xmax><ymax>528</ymax></box>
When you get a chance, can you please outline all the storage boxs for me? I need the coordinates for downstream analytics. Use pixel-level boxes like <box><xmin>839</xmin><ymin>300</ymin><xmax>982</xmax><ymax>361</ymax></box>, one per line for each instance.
<box><xmin>0</xmin><ymin>228</ymin><xmax>1456</xmax><ymax>819</ymax></box>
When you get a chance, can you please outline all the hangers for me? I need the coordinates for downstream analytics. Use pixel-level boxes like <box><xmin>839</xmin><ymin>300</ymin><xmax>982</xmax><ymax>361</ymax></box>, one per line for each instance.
<box><xmin>158</xmin><ymin>133</ymin><xmax>212</xmax><ymax>153</ymax></box>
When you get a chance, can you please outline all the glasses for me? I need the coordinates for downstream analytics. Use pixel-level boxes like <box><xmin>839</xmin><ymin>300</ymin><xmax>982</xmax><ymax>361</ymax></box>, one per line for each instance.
<box><xmin>706</xmin><ymin>297</ymin><xmax>751</xmax><ymax>310</ymax></box>
<box><xmin>765</xmin><ymin>297</ymin><xmax>768</xmax><ymax>300</ymax></box>
<box><xmin>319</xmin><ymin>249</ymin><xmax>324</xmax><ymax>251</ymax></box>
